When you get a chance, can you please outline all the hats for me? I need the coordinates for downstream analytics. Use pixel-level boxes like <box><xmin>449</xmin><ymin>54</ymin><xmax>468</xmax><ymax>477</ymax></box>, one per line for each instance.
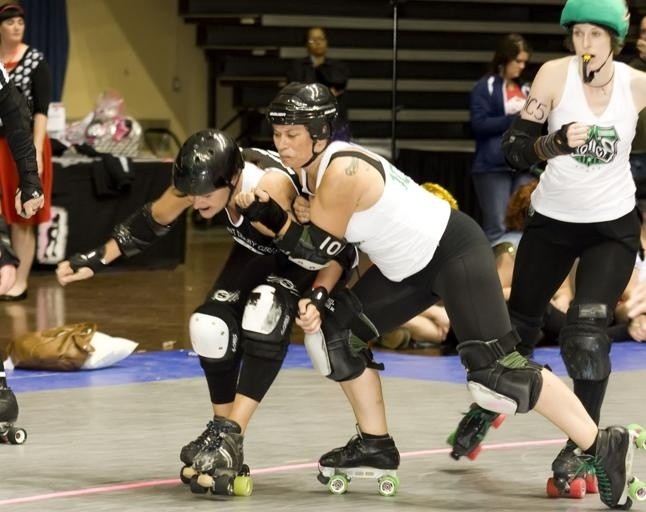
<box><xmin>1</xmin><ymin>4</ymin><xmax>26</xmax><ymax>26</ymax></box>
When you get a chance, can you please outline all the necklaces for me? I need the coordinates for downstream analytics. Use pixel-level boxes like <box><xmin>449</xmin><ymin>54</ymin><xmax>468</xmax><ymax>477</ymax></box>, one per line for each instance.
<box><xmin>582</xmin><ymin>73</ymin><xmax>614</xmax><ymax>89</ymax></box>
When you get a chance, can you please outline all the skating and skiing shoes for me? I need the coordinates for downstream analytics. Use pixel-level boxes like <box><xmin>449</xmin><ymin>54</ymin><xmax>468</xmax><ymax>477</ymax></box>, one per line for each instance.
<box><xmin>1</xmin><ymin>385</ymin><xmax>28</xmax><ymax>446</ymax></box>
<box><xmin>190</xmin><ymin>421</ymin><xmax>253</xmax><ymax>500</ymax></box>
<box><xmin>316</xmin><ymin>434</ymin><xmax>401</xmax><ymax>497</ymax></box>
<box><xmin>545</xmin><ymin>441</ymin><xmax>597</xmax><ymax>496</ymax></box>
<box><xmin>177</xmin><ymin>416</ymin><xmax>222</xmax><ymax>484</ymax></box>
<box><xmin>447</xmin><ymin>402</ymin><xmax>505</xmax><ymax>463</ymax></box>
<box><xmin>573</xmin><ymin>426</ymin><xmax>645</xmax><ymax>509</ymax></box>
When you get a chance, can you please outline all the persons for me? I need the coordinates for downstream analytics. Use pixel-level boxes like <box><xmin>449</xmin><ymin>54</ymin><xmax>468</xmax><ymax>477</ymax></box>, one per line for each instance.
<box><xmin>0</xmin><ymin>61</ymin><xmax>47</xmax><ymax>432</ymax></box>
<box><xmin>465</xmin><ymin>34</ymin><xmax>549</xmax><ymax>245</ymax></box>
<box><xmin>626</xmin><ymin>14</ymin><xmax>646</xmax><ymax>218</ymax></box>
<box><xmin>52</xmin><ymin>126</ymin><xmax>362</xmax><ymax>477</ymax></box>
<box><xmin>443</xmin><ymin>0</ymin><xmax>646</xmax><ymax>495</ymax></box>
<box><xmin>0</xmin><ymin>3</ymin><xmax>53</xmax><ymax>303</ymax></box>
<box><xmin>295</xmin><ymin>26</ymin><xmax>355</xmax><ymax>143</ymax></box>
<box><xmin>234</xmin><ymin>78</ymin><xmax>645</xmax><ymax>511</ymax></box>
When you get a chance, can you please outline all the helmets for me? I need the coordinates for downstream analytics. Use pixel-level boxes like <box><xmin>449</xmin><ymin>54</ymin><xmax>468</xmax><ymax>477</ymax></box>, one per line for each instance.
<box><xmin>266</xmin><ymin>80</ymin><xmax>340</xmax><ymax>140</ymax></box>
<box><xmin>172</xmin><ymin>128</ymin><xmax>246</xmax><ymax>197</ymax></box>
<box><xmin>559</xmin><ymin>0</ymin><xmax>632</xmax><ymax>44</ymax></box>
